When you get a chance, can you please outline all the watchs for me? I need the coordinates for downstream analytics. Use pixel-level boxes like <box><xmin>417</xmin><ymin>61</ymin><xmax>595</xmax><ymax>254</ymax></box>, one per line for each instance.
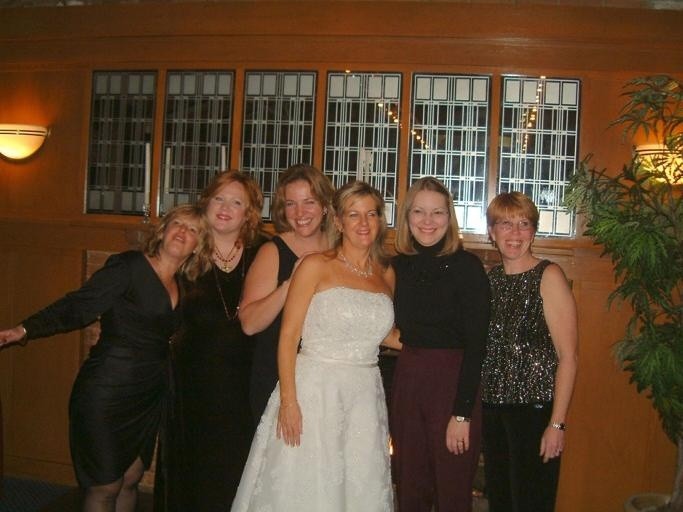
<box><xmin>454</xmin><ymin>414</ymin><xmax>471</xmax><ymax>422</ymax></box>
<box><xmin>548</xmin><ymin>419</ymin><xmax>569</xmax><ymax>432</ymax></box>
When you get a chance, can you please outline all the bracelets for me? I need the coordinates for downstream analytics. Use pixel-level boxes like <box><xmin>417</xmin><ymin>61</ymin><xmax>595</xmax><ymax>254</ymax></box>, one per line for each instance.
<box><xmin>280</xmin><ymin>400</ymin><xmax>298</xmax><ymax>406</ymax></box>
<box><xmin>20</xmin><ymin>327</ymin><xmax>27</xmax><ymax>347</ymax></box>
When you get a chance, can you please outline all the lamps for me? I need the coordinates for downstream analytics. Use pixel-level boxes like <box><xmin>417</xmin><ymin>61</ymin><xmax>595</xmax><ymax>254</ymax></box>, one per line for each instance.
<box><xmin>0</xmin><ymin>122</ymin><xmax>51</xmax><ymax>163</ymax></box>
<box><xmin>628</xmin><ymin>138</ymin><xmax>682</xmax><ymax>187</ymax></box>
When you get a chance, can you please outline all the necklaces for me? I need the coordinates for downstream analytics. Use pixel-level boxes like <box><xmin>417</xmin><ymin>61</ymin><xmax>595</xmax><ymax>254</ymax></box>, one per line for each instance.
<box><xmin>206</xmin><ymin>253</ymin><xmax>250</xmax><ymax>322</ymax></box>
<box><xmin>336</xmin><ymin>245</ymin><xmax>374</xmax><ymax>281</ymax></box>
<box><xmin>212</xmin><ymin>238</ymin><xmax>241</xmax><ymax>271</ymax></box>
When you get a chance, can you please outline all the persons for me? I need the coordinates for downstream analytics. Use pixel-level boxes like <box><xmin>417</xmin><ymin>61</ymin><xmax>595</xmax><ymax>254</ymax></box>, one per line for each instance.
<box><xmin>228</xmin><ymin>178</ymin><xmax>399</xmax><ymax>512</ymax></box>
<box><xmin>147</xmin><ymin>169</ymin><xmax>269</xmax><ymax>511</ymax></box>
<box><xmin>384</xmin><ymin>175</ymin><xmax>491</xmax><ymax>512</ymax></box>
<box><xmin>237</xmin><ymin>162</ymin><xmax>341</xmax><ymax>423</ymax></box>
<box><xmin>481</xmin><ymin>191</ymin><xmax>579</xmax><ymax>511</ymax></box>
<box><xmin>0</xmin><ymin>202</ymin><xmax>216</xmax><ymax>511</ymax></box>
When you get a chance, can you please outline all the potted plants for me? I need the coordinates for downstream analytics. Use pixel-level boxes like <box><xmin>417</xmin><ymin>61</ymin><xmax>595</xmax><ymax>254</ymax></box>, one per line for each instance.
<box><xmin>551</xmin><ymin>66</ymin><xmax>682</xmax><ymax>512</ymax></box>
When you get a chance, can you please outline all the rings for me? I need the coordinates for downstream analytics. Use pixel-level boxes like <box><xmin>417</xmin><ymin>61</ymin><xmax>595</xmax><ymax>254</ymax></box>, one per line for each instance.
<box><xmin>458</xmin><ymin>440</ymin><xmax>463</xmax><ymax>442</ymax></box>
